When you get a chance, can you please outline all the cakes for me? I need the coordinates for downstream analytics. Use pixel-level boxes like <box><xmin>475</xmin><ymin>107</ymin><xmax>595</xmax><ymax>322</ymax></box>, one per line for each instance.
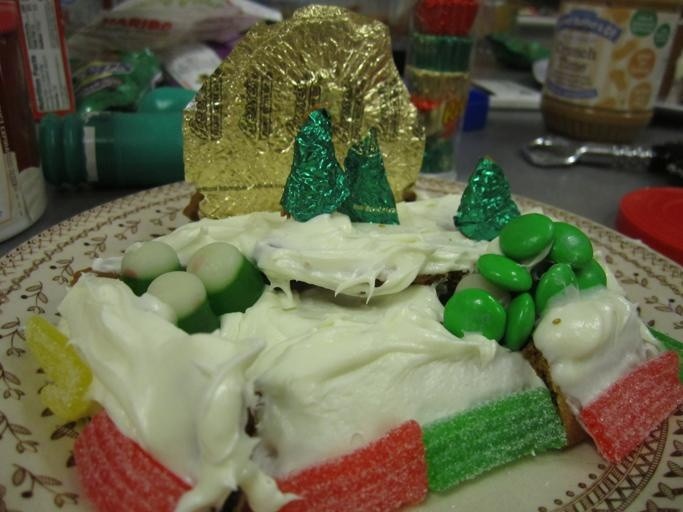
<box><xmin>25</xmin><ymin>107</ymin><xmax>683</xmax><ymax>512</ymax></box>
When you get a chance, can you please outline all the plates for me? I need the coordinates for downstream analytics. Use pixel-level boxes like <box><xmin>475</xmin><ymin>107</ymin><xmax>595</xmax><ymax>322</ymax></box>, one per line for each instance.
<box><xmin>0</xmin><ymin>175</ymin><xmax>682</xmax><ymax>507</ymax></box>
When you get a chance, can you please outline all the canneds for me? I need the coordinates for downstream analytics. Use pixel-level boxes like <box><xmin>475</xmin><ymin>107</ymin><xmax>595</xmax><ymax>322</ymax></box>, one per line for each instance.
<box><xmin>541</xmin><ymin>1</ymin><xmax>682</xmax><ymax>142</ymax></box>
